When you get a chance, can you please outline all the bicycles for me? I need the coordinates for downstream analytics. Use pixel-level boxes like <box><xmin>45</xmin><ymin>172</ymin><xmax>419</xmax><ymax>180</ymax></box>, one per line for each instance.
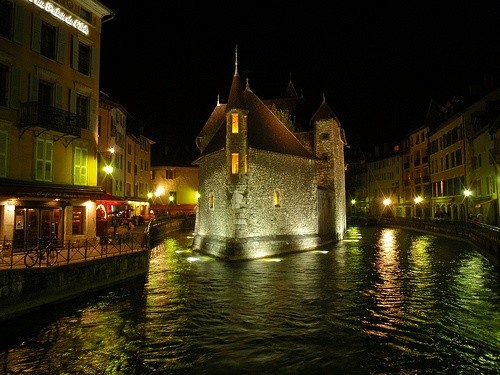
<box><xmin>24</xmin><ymin>236</ymin><xmax>58</xmax><ymax>268</ymax></box>
